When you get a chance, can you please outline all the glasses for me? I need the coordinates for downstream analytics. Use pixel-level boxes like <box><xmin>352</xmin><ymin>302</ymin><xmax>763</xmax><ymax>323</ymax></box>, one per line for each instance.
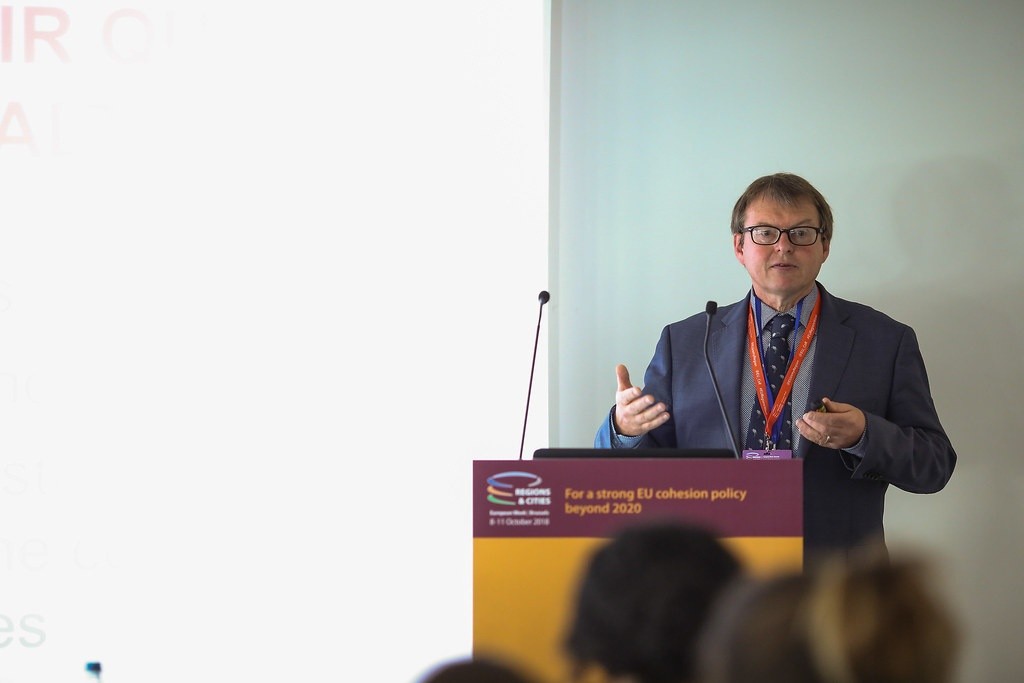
<box><xmin>740</xmin><ymin>225</ymin><xmax>824</xmax><ymax>246</ymax></box>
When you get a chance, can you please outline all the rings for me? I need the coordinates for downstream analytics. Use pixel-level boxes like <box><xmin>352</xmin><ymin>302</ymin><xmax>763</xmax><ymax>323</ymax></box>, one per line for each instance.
<box><xmin>824</xmin><ymin>436</ymin><xmax>830</xmax><ymax>445</ymax></box>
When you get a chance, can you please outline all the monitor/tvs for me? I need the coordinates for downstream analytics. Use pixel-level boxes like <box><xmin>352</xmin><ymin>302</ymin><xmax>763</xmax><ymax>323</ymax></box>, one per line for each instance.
<box><xmin>532</xmin><ymin>447</ymin><xmax>739</xmax><ymax>458</ymax></box>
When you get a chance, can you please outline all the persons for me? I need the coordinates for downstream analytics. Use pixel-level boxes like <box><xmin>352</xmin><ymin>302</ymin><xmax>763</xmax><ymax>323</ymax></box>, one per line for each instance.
<box><xmin>593</xmin><ymin>174</ymin><xmax>957</xmax><ymax>576</ymax></box>
<box><xmin>418</xmin><ymin>526</ymin><xmax>959</xmax><ymax>682</ymax></box>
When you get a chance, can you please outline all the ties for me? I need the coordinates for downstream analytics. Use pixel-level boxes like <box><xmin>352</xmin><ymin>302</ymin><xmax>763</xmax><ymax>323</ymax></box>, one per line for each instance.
<box><xmin>744</xmin><ymin>313</ymin><xmax>802</xmax><ymax>450</ymax></box>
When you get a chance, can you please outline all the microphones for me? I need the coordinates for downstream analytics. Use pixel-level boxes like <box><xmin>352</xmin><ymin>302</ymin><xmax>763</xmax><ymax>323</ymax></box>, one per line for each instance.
<box><xmin>704</xmin><ymin>301</ymin><xmax>739</xmax><ymax>459</ymax></box>
<box><xmin>520</xmin><ymin>290</ymin><xmax>550</xmax><ymax>460</ymax></box>
